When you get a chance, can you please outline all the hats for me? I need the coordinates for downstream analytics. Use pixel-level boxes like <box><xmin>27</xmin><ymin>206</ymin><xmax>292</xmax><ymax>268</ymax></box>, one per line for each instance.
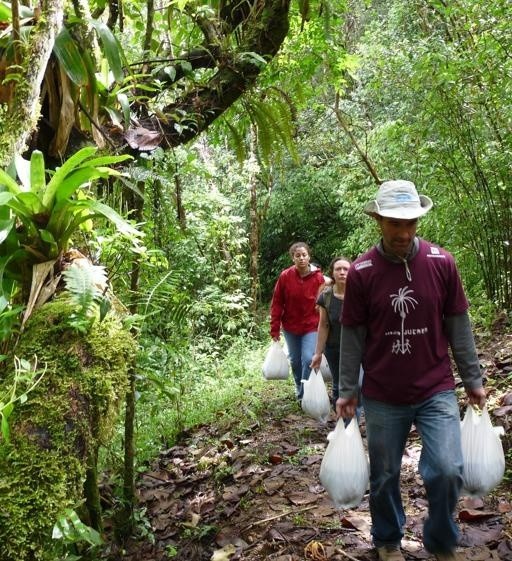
<box><xmin>363</xmin><ymin>179</ymin><xmax>433</xmax><ymax>221</ymax></box>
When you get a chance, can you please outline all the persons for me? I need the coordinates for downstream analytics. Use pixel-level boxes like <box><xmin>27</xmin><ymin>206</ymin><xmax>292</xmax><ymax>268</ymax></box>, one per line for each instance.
<box><xmin>306</xmin><ymin>256</ymin><xmax>364</xmax><ymax>428</ymax></box>
<box><xmin>333</xmin><ymin>175</ymin><xmax>488</xmax><ymax>561</ymax></box>
<box><xmin>311</xmin><ymin>263</ymin><xmax>333</xmax><ymax>285</ymax></box>
<box><xmin>270</xmin><ymin>239</ymin><xmax>326</xmax><ymax>404</ymax></box>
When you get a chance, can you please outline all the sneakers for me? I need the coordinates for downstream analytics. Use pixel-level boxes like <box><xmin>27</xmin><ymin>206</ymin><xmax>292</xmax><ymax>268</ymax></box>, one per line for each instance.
<box><xmin>434</xmin><ymin>548</ymin><xmax>467</xmax><ymax>561</ymax></box>
<box><xmin>375</xmin><ymin>544</ymin><xmax>406</xmax><ymax>561</ymax></box>
